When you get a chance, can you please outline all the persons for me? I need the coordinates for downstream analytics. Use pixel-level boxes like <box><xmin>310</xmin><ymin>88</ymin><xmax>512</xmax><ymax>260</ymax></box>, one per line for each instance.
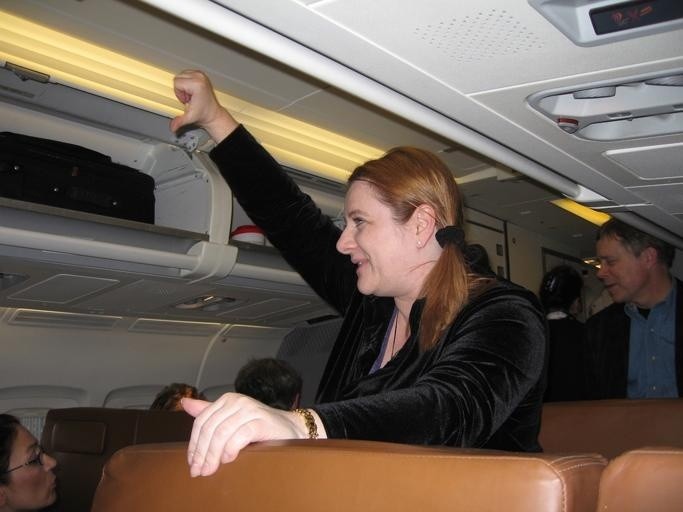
<box><xmin>581</xmin><ymin>217</ymin><xmax>683</xmax><ymax>399</ymax></box>
<box><xmin>170</xmin><ymin>67</ymin><xmax>550</xmax><ymax>479</ymax></box>
<box><xmin>235</xmin><ymin>357</ymin><xmax>303</xmax><ymax>411</ymax></box>
<box><xmin>0</xmin><ymin>411</ymin><xmax>58</xmax><ymax>512</ymax></box>
<box><xmin>151</xmin><ymin>382</ymin><xmax>209</xmax><ymax>411</ymax></box>
<box><xmin>537</xmin><ymin>265</ymin><xmax>593</xmax><ymax>401</ymax></box>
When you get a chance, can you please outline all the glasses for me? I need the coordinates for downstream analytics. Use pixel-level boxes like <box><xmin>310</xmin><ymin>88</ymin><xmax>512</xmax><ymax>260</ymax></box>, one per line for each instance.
<box><xmin>3</xmin><ymin>443</ymin><xmax>47</xmax><ymax>476</ymax></box>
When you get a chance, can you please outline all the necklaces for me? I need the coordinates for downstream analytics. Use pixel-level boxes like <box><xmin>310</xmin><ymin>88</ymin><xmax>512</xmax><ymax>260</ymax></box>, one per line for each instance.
<box><xmin>391</xmin><ymin>310</ymin><xmax>401</xmax><ymax>360</ymax></box>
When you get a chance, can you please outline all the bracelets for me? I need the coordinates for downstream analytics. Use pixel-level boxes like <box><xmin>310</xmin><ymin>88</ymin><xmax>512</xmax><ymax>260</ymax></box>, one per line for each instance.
<box><xmin>293</xmin><ymin>408</ymin><xmax>318</xmax><ymax>441</ymax></box>
